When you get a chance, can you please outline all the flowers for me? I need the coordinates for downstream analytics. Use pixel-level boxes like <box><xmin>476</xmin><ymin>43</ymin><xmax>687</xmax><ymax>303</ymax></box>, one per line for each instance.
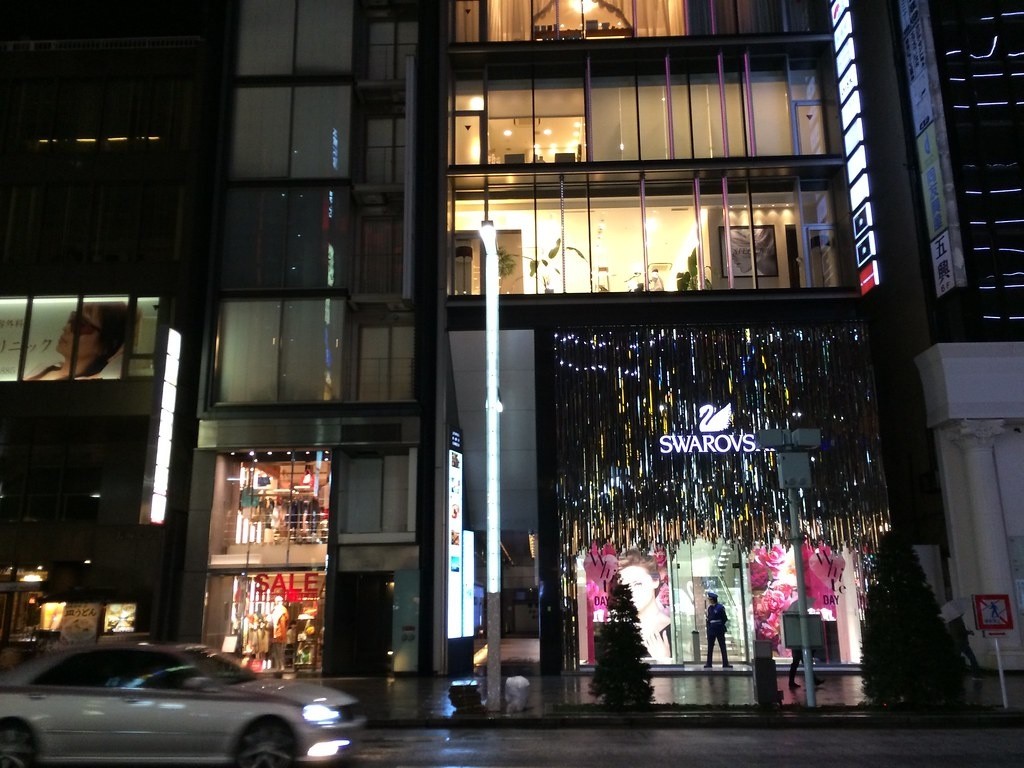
<box><xmin>654</xmin><ymin>548</ymin><xmax>670</xmax><ymax>609</ymax></box>
<box><xmin>747</xmin><ymin>546</ymin><xmax>797</xmax><ymax>657</ymax></box>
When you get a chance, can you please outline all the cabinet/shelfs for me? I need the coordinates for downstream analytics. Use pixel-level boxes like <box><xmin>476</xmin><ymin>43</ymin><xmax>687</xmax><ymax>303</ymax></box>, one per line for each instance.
<box><xmin>294</xmin><ymin>619</ymin><xmax>321</xmax><ymax>672</ymax></box>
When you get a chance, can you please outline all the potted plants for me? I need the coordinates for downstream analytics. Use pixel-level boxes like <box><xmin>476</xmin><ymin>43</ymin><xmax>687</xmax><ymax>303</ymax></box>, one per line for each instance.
<box><xmin>497</xmin><ymin>239</ymin><xmax>588</xmax><ymax>293</ymax></box>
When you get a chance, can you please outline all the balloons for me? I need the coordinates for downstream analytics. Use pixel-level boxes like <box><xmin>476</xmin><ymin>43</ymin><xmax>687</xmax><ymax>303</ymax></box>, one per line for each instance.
<box><xmin>584</xmin><ymin>554</ymin><xmax>619</xmax><ymax>590</ymax></box>
<box><xmin>809</xmin><ymin>552</ymin><xmax>846</xmax><ymax>589</ymax></box>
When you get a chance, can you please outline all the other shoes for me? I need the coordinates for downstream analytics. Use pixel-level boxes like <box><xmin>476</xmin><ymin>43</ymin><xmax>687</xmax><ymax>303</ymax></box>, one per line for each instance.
<box><xmin>704</xmin><ymin>664</ymin><xmax>712</xmax><ymax>668</ymax></box>
<box><xmin>815</xmin><ymin>678</ymin><xmax>825</xmax><ymax>686</ymax></box>
<box><xmin>722</xmin><ymin>664</ymin><xmax>734</xmax><ymax>668</ymax></box>
<box><xmin>789</xmin><ymin>683</ymin><xmax>801</xmax><ymax>689</ymax></box>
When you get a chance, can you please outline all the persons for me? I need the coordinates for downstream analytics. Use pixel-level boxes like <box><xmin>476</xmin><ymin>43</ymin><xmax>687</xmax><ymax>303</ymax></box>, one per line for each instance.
<box><xmin>650</xmin><ymin>267</ymin><xmax>664</xmax><ymax>290</ymax></box>
<box><xmin>629</xmin><ymin>271</ymin><xmax>644</xmax><ymax>291</ymax></box>
<box><xmin>704</xmin><ymin>593</ymin><xmax>733</xmax><ymax>668</ymax></box>
<box><xmin>272</xmin><ymin>596</ymin><xmax>287</xmax><ymax>671</ymax></box>
<box><xmin>789</xmin><ymin>612</ymin><xmax>824</xmax><ymax>688</ymax></box>
<box><xmin>608</xmin><ymin>548</ymin><xmax>672</xmax><ymax>657</ymax></box>
<box><xmin>532</xmin><ymin>155</ymin><xmax>546</xmax><ymax>163</ymax></box>
<box><xmin>285</xmin><ymin>621</ymin><xmax>296</xmax><ymax>665</ymax></box>
<box><xmin>24</xmin><ymin>302</ymin><xmax>128</xmax><ymax>381</ymax></box>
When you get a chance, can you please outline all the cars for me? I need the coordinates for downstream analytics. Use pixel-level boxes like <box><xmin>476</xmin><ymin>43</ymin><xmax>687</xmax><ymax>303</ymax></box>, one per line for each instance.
<box><xmin>0</xmin><ymin>645</ymin><xmax>366</xmax><ymax>768</ymax></box>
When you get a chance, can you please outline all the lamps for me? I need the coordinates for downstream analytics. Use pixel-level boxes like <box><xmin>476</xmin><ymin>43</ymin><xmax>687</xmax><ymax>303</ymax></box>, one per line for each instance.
<box><xmin>455</xmin><ymin>246</ymin><xmax>472</xmax><ymax>295</ymax></box>
<box><xmin>514</xmin><ymin>117</ymin><xmax>541</xmax><ymax>128</ymax></box>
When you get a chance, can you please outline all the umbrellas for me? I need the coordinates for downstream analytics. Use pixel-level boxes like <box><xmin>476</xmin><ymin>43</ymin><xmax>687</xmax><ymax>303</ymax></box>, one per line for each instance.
<box><xmin>785</xmin><ymin>597</ymin><xmax>817</xmax><ymax>614</ymax></box>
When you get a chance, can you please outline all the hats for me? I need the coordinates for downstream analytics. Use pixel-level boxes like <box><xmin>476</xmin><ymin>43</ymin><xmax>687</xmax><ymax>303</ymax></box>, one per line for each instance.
<box><xmin>708</xmin><ymin>593</ymin><xmax>718</xmax><ymax>600</ymax></box>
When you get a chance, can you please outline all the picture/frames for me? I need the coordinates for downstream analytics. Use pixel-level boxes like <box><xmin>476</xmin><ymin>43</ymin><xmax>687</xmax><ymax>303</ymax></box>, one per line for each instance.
<box><xmin>719</xmin><ymin>224</ymin><xmax>779</xmax><ymax>278</ymax></box>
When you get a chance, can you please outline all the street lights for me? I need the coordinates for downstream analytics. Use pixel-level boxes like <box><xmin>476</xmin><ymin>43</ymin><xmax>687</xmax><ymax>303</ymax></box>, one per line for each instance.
<box><xmin>485</xmin><ymin>218</ymin><xmax>502</xmax><ymax>715</ymax></box>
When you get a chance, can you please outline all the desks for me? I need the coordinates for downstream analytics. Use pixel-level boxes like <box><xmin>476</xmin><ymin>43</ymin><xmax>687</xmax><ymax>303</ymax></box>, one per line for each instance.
<box><xmin>534</xmin><ymin>28</ymin><xmax>634</xmax><ymax>41</ymax></box>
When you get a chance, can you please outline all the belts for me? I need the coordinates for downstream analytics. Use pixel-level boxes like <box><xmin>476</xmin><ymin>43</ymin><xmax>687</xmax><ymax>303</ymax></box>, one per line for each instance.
<box><xmin>710</xmin><ymin>619</ymin><xmax>722</xmax><ymax>623</ymax></box>
